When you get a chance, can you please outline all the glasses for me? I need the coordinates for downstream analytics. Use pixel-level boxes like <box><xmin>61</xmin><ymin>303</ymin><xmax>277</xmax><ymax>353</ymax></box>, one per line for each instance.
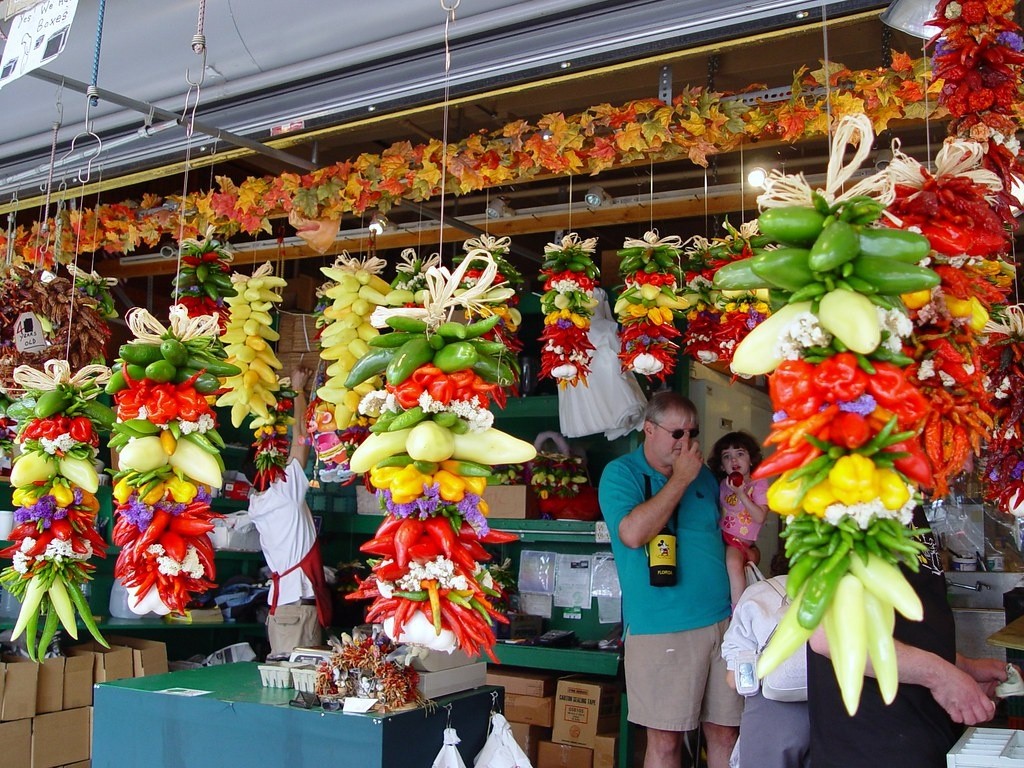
<box><xmin>647</xmin><ymin>419</ymin><xmax>699</xmax><ymax>439</ymax></box>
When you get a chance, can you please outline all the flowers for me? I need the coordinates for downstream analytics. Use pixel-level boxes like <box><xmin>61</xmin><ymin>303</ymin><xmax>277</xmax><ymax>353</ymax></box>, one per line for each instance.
<box><xmin>0</xmin><ymin>0</ymin><xmax>1024</xmax><ymax>668</ymax></box>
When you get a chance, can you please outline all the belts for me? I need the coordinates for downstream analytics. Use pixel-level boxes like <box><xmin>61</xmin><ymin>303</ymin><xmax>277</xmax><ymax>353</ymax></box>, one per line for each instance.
<box><xmin>287</xmin><ymin>598</ymin><xmax>316</xmax><ymax>606</ymax></box>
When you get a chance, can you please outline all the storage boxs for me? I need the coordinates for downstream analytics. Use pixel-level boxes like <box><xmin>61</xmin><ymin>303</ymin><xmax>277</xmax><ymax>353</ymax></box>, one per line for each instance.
<box><xmin>257</xmin><ymin>661</ymin><xmax>486</xmax><ymax>699</ymax></box>
<box><xmin>486</xmin><ymin>669</ymin><xmax>620</xmax><ymax>768</ymax></box>
<box><xmin>0</xmin><ymin>634</ymin><xmax>168</xmax><ymax>768</ymax></box>
<box><xmin>483</xmin><ymin>484</ymin><xmax>527</xmax><ymax>519</ymax></box>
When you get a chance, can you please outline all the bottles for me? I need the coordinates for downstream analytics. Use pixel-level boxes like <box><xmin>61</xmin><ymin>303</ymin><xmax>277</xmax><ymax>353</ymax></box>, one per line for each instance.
<box><xmin>648</xmin><ymin>525</ymin><xmax>677</xmax><ymax>587</ymax></box>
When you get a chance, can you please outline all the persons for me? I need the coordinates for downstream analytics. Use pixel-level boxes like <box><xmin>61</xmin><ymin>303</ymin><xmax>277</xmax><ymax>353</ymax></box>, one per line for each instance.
<box><xmin>721</xmin><ymin>551</ymin><xmax>812</xmax><ymax>767</ymax></box>
<box><xmin>807</xmin><ymin>494</ymin><xmax>1020</xmax><ymax>767</ymax></box>
<box><xmin>247</xmin><ymin>362</ymin><xmax>331</xmax><ymax>655</ymax></box>
<box><xmin>598</xmin><ymin>390</ymin><xmax>761</xmax><ymax>767</ymax></box>
<box><xmin>706</xmin><ymin>430</ymin><xmax>772</xmax><ymax>619</ymax></box>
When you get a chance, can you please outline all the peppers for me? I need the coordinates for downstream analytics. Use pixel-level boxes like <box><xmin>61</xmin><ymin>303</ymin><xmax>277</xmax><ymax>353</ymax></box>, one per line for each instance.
<box><xmin>0</xmin><ymin>242</ymin><xmax>536</xmax><ymax>707</ymax></box>
<box><xmin>491</xmin><ymin>452</ymin><xmax>588</xmax><ymax>500</ymax></box>
<box><xmin>535</xmin><ymin>215</ymin><xmax>776</xmax><ymax>384</ymax></box>
<box><xmin>724</xmin><ymin>2</ymin><xmax>1024</xmax><ymax>717</ymax></box>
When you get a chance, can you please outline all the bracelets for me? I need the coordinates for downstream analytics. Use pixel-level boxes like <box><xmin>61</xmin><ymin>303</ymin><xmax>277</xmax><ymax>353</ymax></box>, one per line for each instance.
<box><xmin>294</xmin><ymin>389</ymin><xmax>306</xmax><ymax>394</ymax></box>
